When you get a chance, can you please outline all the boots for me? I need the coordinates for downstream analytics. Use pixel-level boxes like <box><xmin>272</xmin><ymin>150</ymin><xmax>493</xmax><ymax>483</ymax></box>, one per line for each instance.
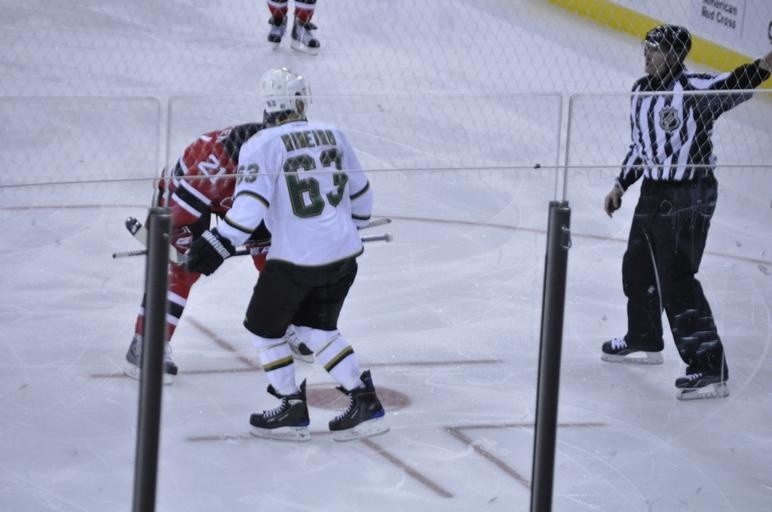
<box><xmin>286</xmin><ymin>330</ymin><xmax>313</xmax><ymax>356</ymax></box>
<box><xmin>250</xmin><ymin>379</ymin><xmax>310</xmax><ymax>428</ymax></box>
<box><xmin>126</xmin><ymin>334</ymin><xmax>178</xmax><ymax>375</ymax></box>
<box><xmin>292</xmin><ymin>20</ymin><xmax>320</xmax><ymax>47</ymax></box>
<box><xmin>329</xmin><ymin>369</ymin><xmax>385</xmax><ymax>431</ymax></box>
<box><xmin>268</xmin><ymin>16</ymin><xmax>288</xmax><ymax>42</ymax></box>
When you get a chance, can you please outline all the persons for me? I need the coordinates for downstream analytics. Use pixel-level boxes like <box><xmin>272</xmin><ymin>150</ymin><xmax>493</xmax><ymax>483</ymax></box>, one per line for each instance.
<box><xmin>185</xmin><ymin>67</ymin><xmax>386</xmax><ymax>431</ymax></box>
<box><xmin>600</xmin><ymin>24</ymin><xmax>771</xmax><ymax>391</ymax></box>
<box><xmin>264</xmin><ymin>1</ymin><xmax>323</xmax><ymax>49</ymax></box>
<box><xmin>125</xmin><ymin>121</ymin><xmax>313</xmax><ymax>378</ymax></box>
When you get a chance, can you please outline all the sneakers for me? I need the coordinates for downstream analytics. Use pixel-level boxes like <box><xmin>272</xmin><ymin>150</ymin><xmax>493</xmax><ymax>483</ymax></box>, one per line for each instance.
<box><xmin>675</xmin><ymin>373</ymin><xmax>728</xmax><ymax>387</ymax></box>
<box><xmin>602</xmin><ymin>335</ymin><xmax>664</xmax><ymax>355</ymax></box>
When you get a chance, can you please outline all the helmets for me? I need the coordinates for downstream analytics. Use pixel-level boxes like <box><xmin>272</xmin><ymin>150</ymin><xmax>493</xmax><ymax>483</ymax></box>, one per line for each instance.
<box><xmin>261</xmin><ymin>68</ymin><xmax>308</xmax><ymax>116</ymax></box>
<box><xmin>646</xmin><ymin>25</ymin><xmax>691</xmax><ymax>51</ymax></box>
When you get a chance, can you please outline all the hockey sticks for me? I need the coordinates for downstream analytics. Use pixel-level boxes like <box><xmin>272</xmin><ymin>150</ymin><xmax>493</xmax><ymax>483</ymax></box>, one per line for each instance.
<box><xmin>113</xmin><ymin>218</ymin><xmax>393</xmax><ymax>257</ymax></box>
<box><xmin>123</xmin><ymin>217</ymin><xmax>390</xmax><ymax>264</ymax></box>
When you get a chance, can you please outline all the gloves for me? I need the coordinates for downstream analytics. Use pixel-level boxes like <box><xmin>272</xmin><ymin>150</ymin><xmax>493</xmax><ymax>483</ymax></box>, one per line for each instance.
<box><xmin>187</xmin><ymin>228</ymin><xmax>235</xmax><ymax>276</ymax></box>
<box><xmin>605</xmin><ymin>184</ymin><xmax>622</xmax><ymax>218</ymax></box>
